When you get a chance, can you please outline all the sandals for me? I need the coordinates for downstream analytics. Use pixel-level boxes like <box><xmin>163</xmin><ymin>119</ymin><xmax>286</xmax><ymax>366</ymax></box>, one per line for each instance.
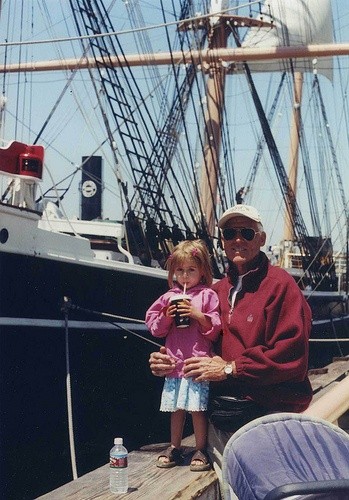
<box><xmin>156</xmin><ymin>447</ymin><xmax>184</xmax><ymax>468</ymax></box>
<box><xmin>189</xmin><ymin>449</ymin><xmax>212</xmax><ymax>471</ymax></box>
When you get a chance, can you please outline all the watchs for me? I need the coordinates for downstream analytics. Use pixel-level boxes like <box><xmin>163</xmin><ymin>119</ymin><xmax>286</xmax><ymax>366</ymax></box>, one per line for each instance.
<box><xmin>224</xmin><ymin>362</ymin><xmax>233</xmax><ymax>379</ymax></box>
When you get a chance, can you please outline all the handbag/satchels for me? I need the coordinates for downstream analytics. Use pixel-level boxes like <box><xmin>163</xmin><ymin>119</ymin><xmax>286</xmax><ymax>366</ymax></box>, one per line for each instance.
<box><xmin>209</xmin><ymin>388</ymin><xmax>268</xmax><ymax>432</ymax></box>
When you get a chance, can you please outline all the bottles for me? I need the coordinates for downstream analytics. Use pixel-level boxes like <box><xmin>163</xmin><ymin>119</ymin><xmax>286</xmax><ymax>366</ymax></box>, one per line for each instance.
<box><xmin>109</xmin><ymin>437</ymin><xmax>128</xmax><ymax>494</ymax></box>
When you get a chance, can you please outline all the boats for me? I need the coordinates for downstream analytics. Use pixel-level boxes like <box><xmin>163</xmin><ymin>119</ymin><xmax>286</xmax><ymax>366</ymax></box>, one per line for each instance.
<box><xmin>0</xmin><ymin>0</ymin><xmax>349</xmax><ymax>499</ymax></box>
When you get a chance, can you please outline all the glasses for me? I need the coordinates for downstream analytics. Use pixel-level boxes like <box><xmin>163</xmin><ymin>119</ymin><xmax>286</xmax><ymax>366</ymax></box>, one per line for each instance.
<box><xmin>223</xmin><ymin>227</ymin><xmax>261</xmax><ymax>243</ymax></box>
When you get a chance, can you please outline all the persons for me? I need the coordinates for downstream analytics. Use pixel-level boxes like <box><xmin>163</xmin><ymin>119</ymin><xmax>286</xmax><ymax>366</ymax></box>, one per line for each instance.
<box><xmin>148</xmin><ymin>205</ymin><xmax>313</xmax><ymax>500</ymax></box>
<box><xmin>144</xmin><ymin>240</ymin><xmax>223</xmax><ymax>472</ymax></box>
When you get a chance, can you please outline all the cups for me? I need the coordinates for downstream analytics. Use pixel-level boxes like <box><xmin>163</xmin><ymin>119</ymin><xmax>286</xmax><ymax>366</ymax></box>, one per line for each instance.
<box><xmin>169</xmin><ymin>295</ymin><xmax>192</xmax><ymax>328</ymax></box>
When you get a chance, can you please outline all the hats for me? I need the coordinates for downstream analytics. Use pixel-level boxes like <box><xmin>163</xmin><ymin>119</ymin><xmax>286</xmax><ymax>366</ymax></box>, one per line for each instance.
<box><xmin>218</xmin><ymin>204</ymin><xmax>264</xmax><ymax>228</ymax></box>
